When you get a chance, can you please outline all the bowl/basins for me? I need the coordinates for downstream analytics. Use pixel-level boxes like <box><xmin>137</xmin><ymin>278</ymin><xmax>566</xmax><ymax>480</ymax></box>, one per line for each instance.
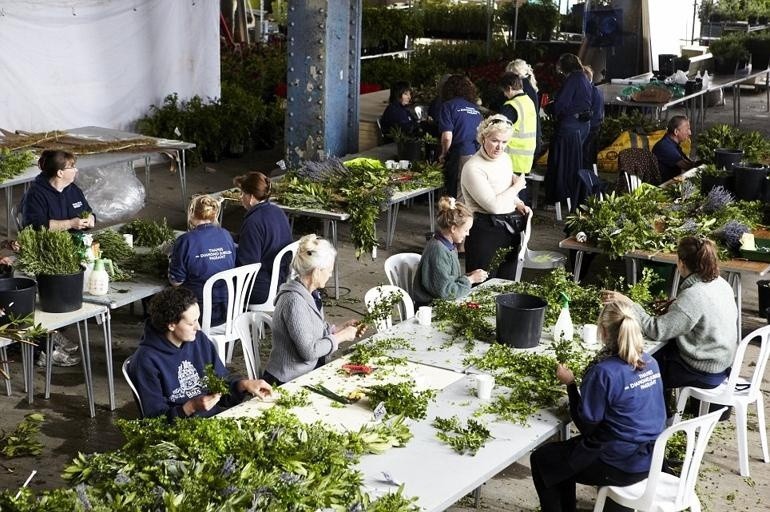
<box><xmin>631</xmin><ymin>97</ymin><xmax>672</xmax><ymax>103</ymax></box>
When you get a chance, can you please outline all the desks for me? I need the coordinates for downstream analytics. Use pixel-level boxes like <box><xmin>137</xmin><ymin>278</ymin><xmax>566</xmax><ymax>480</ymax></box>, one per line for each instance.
<box><xmin>206</xmin><ymin>277</ymin><xmax>670</xmax><ymax>512</ymax></box>
<box><xmin>559</xmin><ymin>52</ymin><xmax>769</xmax><ymax>344</ymax></box>
<box><xmin>0</xmin><ymin>125</ymin><xmax>447</xmax><ymax>424</ymax></box>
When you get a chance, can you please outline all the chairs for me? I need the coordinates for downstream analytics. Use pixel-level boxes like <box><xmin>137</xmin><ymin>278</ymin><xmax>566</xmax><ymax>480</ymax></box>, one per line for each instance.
<box><xmin>672</xmin><ymin>326</ymin><xmax>770</xmax><ymax>476</ymax></box>
<box><xmin>593</xmin><ymin>406</ymin><xmax>729</xmax><ymax>512</ymax></box>
<box><xmin>527</xmin><ymin>165</ymin><xmax>572</xmax><ymax>221</ymax></box>
<box><xmin>514</xmin><ymin>205</ymin><xmax>534</xmax><ymax>281</ymax></box>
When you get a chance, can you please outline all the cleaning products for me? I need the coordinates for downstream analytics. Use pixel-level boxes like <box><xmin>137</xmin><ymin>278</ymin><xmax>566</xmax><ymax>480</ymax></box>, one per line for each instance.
<box><xmin>553</xmin><ymin>290</ymin><xmax>574</xmax><ymax>342</ymax></box>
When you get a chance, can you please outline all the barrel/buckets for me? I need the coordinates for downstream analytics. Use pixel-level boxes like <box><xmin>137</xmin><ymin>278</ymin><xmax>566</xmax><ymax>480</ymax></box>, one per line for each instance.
<box><xmin>701</xmin><ymin>147</ymin><xmax>770</xmax><ymax>204</ymax></box>
<box><xmin>34</xmin><ymin>263</ymin><xmax>87</xmax><ymax>314</ymax></box>
<box><xmin>658</xmin><ymin>54</ymin><xmax>691</xmax><ymax>75</ymax></box>
<box><xmin>0</xmin><ymin>264</ymin><xmax>15</xmax><ymax>279</ymax></box>
<box><xmin>255</xmin><ymin>20</ymin><xmax>280</xmax><ymax>44</ymax></box>
<box><xmin>756</xmin><ymin>279</ymin><xmax>770</xmax><ymax>320</ymax></box>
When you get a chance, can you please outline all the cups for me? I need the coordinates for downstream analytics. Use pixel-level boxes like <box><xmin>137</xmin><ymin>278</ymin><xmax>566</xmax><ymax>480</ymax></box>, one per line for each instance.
<box><xmin>122</xmin><ymin>234</ymin><xmax>134</xmax><ymax>248</ymax></box>
<box><xmin>478</xmin><ymin>374</ymin><xmax>495</xmax><ymax>400</ymax></box>
<box><xmin>715</xmin><ymin>148</ymin><xmax>769</xmax><ymax>200</ymax></box>
<box><xmin>577</xmin><ymin>324</ymin><xmax>597</xmax><ymax>345</ymax></box>
<box><xmin>385</xmin><ymin>160</ymin><xmax>413</xmax><ymax>171</ymax></box>
<box><xmin>745</xmin><ymin>64</ymin><xmax>752</xmax><ymax>75</ymax></box>
<box><xmin>756</xmin><ymin>280</ymin><xmax>770</xmax><ymax>317</ymax></box>
<box><xmin>416</xmin><ymin>306</ymin><xmax>433</xmax><ymax>325</ymax></box>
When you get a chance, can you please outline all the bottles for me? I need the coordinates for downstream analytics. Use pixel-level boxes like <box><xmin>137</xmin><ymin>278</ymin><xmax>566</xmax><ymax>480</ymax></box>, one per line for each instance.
<box><xmin>623</xmin><ymin>68</ymin><xmax>710</xmax><ymax>97</ymax></box>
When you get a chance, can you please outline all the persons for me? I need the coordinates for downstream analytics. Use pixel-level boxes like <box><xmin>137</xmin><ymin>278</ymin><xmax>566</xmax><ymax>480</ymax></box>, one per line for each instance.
<box><xmin>128</xmin><ymin>286</ymin><xmax>275</xmax><ymax>424</ymax></box>
<box><xmin>167</xmin><ymin>194</ymin><xmax>236</xmax><ymax>328</ymax></box>
<box><xmin>652</xmin><ymin>114</ymin><xmax>709</xmax><ymax>185</ymax></box>
<box><xmin>580</xmin><ymin>64</ymin><xmax>606</xmax><ymax>208</ymax></box>
<box><xmin>544</xmin><ymin>51</ymin><xmax>593</xmax><ymax>214</ymax></box>
<box><xmin>19</xmin><ymin>149</ymin><xmax>97</xmax><ymax>368</ymax></box>
<box><xmin>598</xmin><ymin>235</ymin><xmax>739</xmax><ymax>419</ymax></box>
<box><xmin>234</xmin><ymin>170</ymin><xmax>296</xmax><ymax>302</ymax></box>
<box><xmin>262</xmin><ymin>232</ymin><xmax>369</xmax><ymax>388</ymax></box>
<box><xmin>379</xmin><ymin>81</ymin><xmax>419</xmax><ymax>140</ymax></box>
<box><xmin>459</xmin><ymin>112</ymin><xmax>532</xmax><ymax>289</ymax></box>
<box><xmin>411</xmin><ymin>194</ymin><xmax>491</xmax><ymax>316</ymax></box>
<box><xmin>436</xmin><ymin>57</ymin><xmax>545</xmax><ymax>207</ymax></box>
<box><xmin>528</xmin><ymin>300</ymin><xmax>666</xmax><ymax>512</ymax></box>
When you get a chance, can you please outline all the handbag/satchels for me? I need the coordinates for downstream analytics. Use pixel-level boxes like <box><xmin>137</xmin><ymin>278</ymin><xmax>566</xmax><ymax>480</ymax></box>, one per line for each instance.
<box><xmin>475</xmin><ymin>213</ymin><xmax>527</xmax><ymax>234</ymax></box>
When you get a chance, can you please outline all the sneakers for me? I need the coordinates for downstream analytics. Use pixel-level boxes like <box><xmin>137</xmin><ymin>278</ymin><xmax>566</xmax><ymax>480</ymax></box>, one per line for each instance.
<box><xmin>36</xmin><ymin>330</ymin><xmax>81</xmax><ymax>367</ymax></box>
